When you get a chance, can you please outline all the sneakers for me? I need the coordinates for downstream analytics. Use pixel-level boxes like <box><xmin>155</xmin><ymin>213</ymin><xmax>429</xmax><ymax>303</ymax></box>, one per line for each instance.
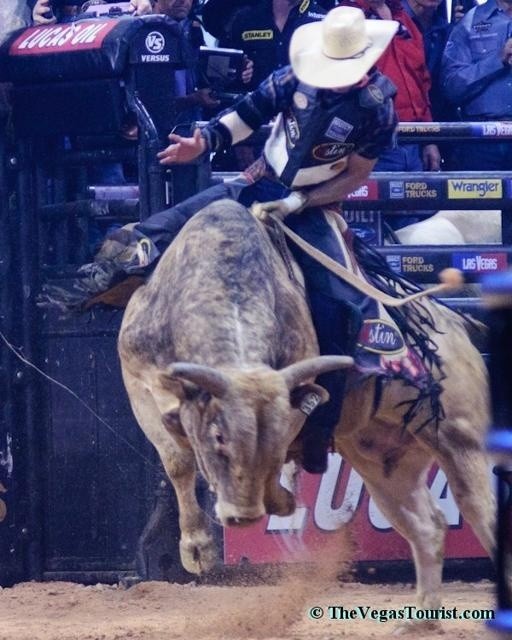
<box><xmin>42</xmin><ymin>284</ymin><xmax>91</xmax><ymax>315</ymax></box>
<box><xmin>301</xmin><ymin>432</ymin><xmax>328</xmax><ymax>475</ymax></box>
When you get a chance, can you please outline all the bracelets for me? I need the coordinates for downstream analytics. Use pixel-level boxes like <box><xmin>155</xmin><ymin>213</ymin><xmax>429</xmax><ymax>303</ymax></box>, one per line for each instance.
<box><xmin>282</xmin><ymin>189</ymin><xmax>303</xmax><ymax>215</ymax></box>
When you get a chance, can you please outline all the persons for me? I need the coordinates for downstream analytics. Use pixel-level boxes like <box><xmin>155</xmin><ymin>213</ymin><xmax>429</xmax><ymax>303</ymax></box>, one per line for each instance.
<box><xmin>74</xmin><ymin>5</ymin><xmax>406</xmax><ymax>474</ymax></box>
<box><xmin>1</xmin><ymin>0</ymin><xmax>512</xmax><ymax>172</ymax></box>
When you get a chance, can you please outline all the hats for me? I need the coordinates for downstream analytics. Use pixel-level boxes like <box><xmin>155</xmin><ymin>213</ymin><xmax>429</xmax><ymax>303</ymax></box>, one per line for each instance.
<box><xmin>288</xmin><ymin>7</ymin><xmax>399</xmax><ymax>88</ymax></box>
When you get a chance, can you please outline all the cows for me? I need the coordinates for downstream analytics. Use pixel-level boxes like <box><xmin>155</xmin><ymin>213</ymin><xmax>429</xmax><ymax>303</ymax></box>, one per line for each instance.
<box><xmin>118</xmin><ymin>198</ymin><xmax>512</xmax><ymax>636</ymax></box>
